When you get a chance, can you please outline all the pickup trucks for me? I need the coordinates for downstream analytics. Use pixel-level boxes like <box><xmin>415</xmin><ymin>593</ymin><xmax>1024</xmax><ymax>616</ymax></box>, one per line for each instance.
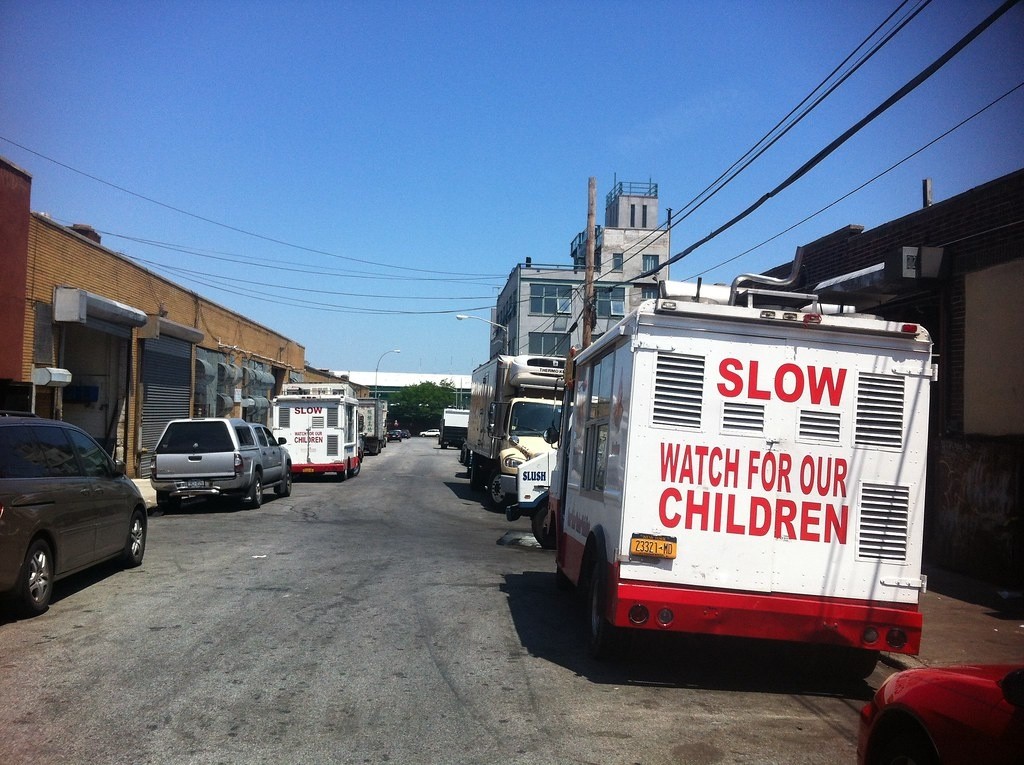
<box><xmin>149</xmin><ymin>417</ymin><xmax>293</xmax><ymax>507</ymax></box>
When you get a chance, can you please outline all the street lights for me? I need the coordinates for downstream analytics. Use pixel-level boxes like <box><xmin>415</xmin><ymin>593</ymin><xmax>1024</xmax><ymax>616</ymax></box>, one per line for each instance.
<box><xmin>375</xmin><ymin>349</ymin><xmax>401</xmax><ymax>399</ymax></box>
<box><xmin>456</xmin><ymin>314</ymin><xmax>509</xmax><ymax>355</ymax></box>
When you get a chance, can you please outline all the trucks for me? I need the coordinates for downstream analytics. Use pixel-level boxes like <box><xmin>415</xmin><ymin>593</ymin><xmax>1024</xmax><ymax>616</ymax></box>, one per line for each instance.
<box><xmin>438</xmin><ymin>409</ymin><xmax>471</xmax><ymax>449</ymax></box>
<box><xmin>469</xmin><ymin>355</ymin><xmax>575</xmax><ymax>515</ymax></box>
<box><xmin>357</xmin><ymin>398</ymin><xmax>388</xmax><ymax>455</ymax></box>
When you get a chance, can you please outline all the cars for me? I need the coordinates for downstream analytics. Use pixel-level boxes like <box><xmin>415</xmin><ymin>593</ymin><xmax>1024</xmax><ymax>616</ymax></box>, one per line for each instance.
<box><xmin>402</xmin><ymin>429</ymin><xmax>411</xmax><ymax>439</ymax></box>
<box><xmin>856</xmin><ymin>663</ymin><xmax>1023</xmax><ymax>765</ymax></box>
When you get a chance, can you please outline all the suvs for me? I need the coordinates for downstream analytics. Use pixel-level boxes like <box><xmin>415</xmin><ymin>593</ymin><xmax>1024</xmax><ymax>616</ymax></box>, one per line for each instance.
<box><xmin>0</xmin><ymin>409</ymin><xmax>148</xmax><ymax>616</ymax></box>
<box><xmin>386</xmin><ymin>430</ymin><xmax>403</xmax><ymax>443</ymax></box>
<box><xmin>420</xmin><ymin>428</ymin><xmax>440</xmax><ymax>437</ymax></box>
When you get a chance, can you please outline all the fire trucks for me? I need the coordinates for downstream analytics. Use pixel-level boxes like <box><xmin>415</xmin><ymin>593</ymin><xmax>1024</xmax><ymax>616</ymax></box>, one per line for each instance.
<box><xmin>542</xmin><ymin>247</ymin><xmax>941</xmax><ymax>689</ymax></box>
<box><xmin>272</xmin><ymin>394</ymin><xmax>362</xmax><ymax>482</ymax></box>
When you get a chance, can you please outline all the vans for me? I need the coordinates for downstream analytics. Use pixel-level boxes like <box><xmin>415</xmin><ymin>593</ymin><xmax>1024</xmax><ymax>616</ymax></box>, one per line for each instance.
<box><xmin>502</xmin><ymin>412</ymin><xmax>577</xmax><ymax>551</ymax></box>
